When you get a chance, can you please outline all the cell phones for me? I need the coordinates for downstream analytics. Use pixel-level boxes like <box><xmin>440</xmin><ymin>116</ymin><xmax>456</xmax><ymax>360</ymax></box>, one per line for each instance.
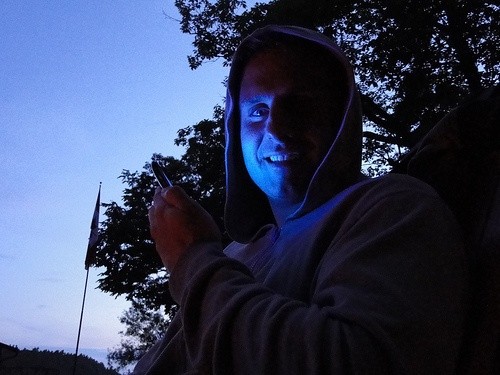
<box><xmin>152</xmin><ymin>161</ymin><xmax>172</xmax><ymax>190</ymax></box>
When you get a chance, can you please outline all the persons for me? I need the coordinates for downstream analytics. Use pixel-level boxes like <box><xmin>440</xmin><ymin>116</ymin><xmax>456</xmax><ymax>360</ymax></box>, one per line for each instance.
<box><xmin>131</xmin><ymin>22</ymin><xmax>470</xmax><ymax>375</ymax></box>
